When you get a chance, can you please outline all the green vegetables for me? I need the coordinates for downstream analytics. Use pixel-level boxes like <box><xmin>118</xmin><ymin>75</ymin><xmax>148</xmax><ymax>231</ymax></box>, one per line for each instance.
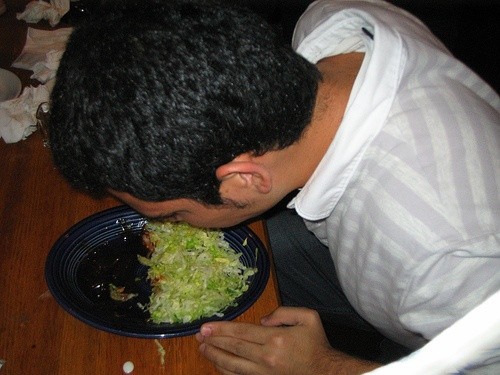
<box><xmin>136</xmin><ymin>219</ymin><xmax>258</xmax><ymax>326</ymax></box>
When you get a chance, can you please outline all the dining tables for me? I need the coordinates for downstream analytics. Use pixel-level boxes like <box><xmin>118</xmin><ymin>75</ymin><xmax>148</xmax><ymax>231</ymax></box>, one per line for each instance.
<box><xmin>0</xmin><ymin>17</ymin><xmax>282</xmax><ymax>374</ymax></box>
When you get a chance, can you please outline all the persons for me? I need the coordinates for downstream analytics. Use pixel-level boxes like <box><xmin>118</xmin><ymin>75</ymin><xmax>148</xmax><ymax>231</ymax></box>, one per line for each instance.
<box><xmin>47</xmin><ymin>0</ymin><xmax>500</xmax><ymax>375</ymax></box>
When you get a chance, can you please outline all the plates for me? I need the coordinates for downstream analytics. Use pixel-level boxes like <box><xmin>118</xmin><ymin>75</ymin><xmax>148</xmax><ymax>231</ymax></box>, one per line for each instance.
<box><xmin>0</xmin><ymin>67</ymin><xmax>21</xmax><ymax>107</ymax></box>
<box><xmin>44</xmin><ymin>203</ymin><xmax>272</xmax><ymax>340</ymax></box>
